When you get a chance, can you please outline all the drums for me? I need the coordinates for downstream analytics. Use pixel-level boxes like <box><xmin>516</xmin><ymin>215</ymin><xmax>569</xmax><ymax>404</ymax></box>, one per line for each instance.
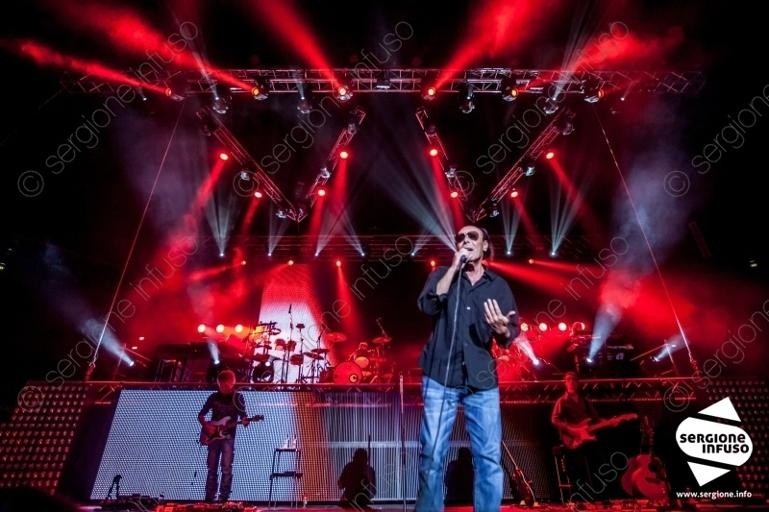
<box><xmin>320</xmin><ymin>350</ymin><xmax>372</xmax><ymax>384</ymax></box>
<box><xmin>287</xmin><ymin>340</ymin><xmax>297</xmax><ymax>351</ymax></box>
<box><xmin>276</xmin><ymin>339</ymin><xmax>285</xmax><ymax>351</ymax></box>
<box><xmin>291</xmin><ymin>354</ymin><xmax>303</xmax><ymax>365</ymax></box>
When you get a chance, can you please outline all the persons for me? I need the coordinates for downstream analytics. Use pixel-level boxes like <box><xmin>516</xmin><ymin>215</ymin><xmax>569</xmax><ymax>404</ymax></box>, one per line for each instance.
<box><xmin>197</xmin><ymin>369</ymin><xmax>249</xmax><ymax>502</ymax></box>
<box><xmin>445</xmin><ymin>445</ymin><xmax>474</xmax><ymax>508</ymax></box>
<box><xmin>639</xmin><ymin>386</ymin><xmax>695</xmax><ymax>512</ymax></box>
<box><xmin>409</xmin><ymin>222</ymin><xmax>521</xmax><ymax>512</ymax></box>
<box><xmin>551</xmin><ymin>369</ymin><xmax>603</xmax><ymax>503</ymax></box>
<box><xmin>336</xmin><ymin>443</ymin><xmax>379</xmax><ymax>508</ymax></box>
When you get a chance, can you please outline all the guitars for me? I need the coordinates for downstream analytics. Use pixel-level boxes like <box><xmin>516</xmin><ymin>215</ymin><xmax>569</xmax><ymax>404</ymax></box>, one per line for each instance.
<box><xmin>201</xmin><ymin>415</ymin><xmax>264</xmax><ymax>445</ymax></box>
<box><xmin>560</xmin><ymin>414</ymin><xmax>638</xmax><ymax>449</ymax></box>
<box><xmin>501</xmin><ymin>439</ymin><xmax>536</xmax><ymax>507</ymax></box>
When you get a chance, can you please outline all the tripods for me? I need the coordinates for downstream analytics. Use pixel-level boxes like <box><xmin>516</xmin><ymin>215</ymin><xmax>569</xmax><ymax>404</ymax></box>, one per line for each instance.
<box><xmin>274</xmin><ymin>313</ymin><xmax>323</xmax><ymax>390</ymax></box>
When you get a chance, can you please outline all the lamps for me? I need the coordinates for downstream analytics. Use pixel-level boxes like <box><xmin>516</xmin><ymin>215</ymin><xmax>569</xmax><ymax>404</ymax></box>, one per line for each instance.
<box><xmin>639</xmin><ymin>355</ymin><xmax>662</xmax><ymax>377</ymax></box>
<box><xmin>126</xmin><ymin>360</ymin><xmax>150</xmax><ymax>379</ymax></box>
<box><xmin>164</xmin><ymin>71</ymin><xmax>606</xmax><ymax>115</ymax></box>
<box><xmin>208</xmin><ymin>357</ymin><xmax>229</xmax><ymax>379</ymax></box>
<box><xmin>532</xmin><ymin>356</ymin><xmax>552</xmax><ymax>380</ymax></box>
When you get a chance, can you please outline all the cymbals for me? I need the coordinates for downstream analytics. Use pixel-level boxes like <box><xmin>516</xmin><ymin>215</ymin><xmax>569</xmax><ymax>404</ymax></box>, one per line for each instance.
<box><xmin>304</xmin><ymin>352</ymin><xmax>325</xmax><ymax>360</ymax></box>
<box><xmin>311</xmin><ymin>348</ymin><xmax>328</xmax><ymax>353</ymax></box>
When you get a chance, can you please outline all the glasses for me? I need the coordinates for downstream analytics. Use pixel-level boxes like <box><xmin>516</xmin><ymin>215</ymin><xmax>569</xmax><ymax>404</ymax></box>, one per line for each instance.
<box><xmin>453</xmin><ymin>230</ymin><xmax>479</xmax><ymax>242</ymax></box>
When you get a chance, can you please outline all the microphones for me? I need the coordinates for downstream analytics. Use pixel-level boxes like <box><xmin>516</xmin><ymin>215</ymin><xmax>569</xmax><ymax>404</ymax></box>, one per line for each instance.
<box><xmin>460</xmin><ymin>248</ymin><xmax>468</xmax><ymax>263</ymax></box>
<box><xmin>288</xmin><ymin>304</ymin><xmax>292</xmax><ymax>313</ymax></box>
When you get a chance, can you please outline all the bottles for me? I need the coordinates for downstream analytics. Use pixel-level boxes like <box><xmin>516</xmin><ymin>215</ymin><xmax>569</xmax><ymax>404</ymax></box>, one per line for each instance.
<box><xmin>292</xmin><ymin>434</ymin><xmax>297</xmax><ymax>449</ymax></box>
<box><xmin>284</xmin><ymin>434</ymin><xmax>290</xmax><ymax>449</ymax></box>
<box><xmin>302</xmin><ymin>496</ymin><xmax>308</xmax><ymax>508</ymax></box>
